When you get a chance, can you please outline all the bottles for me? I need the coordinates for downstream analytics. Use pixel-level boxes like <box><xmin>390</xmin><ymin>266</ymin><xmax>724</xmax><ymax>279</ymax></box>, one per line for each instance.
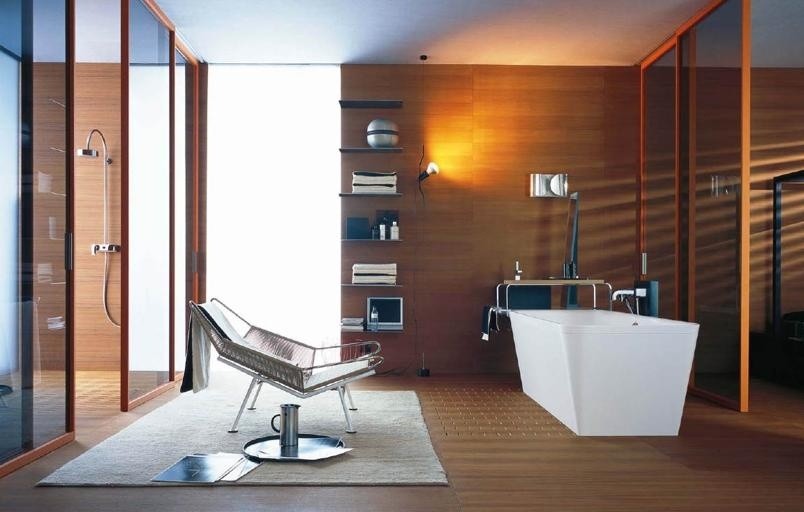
<box><xmin>370</xmin><ymin>305</ymin><xmax>378</xmax><ymax>332</ymax></box>
<box><xmin>515</xmin><ymin>260</ymin><xmax>521</xmax><ymax>280</ymax></box>
<box><xmin>371</xmin><ymin>221</ymin><xmax>399</xmax><ymax>241</ymax></box>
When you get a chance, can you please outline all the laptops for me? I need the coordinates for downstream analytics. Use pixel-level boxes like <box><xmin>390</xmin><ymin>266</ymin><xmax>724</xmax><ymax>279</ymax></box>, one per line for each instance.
<box><xmin>367</xmin><ymin>296</ymin><xmax>404</xmax><ymax>330</ymax></box>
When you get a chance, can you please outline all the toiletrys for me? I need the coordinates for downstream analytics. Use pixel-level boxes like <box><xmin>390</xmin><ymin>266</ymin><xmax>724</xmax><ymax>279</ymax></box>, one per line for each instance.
<box><xmin>389</xmin><ymin>222</ymin><xmax>399</xmax><ymax>241</ymax></box>
<box><xmin>371</xmin><ymin>225</ymin><xmax>378</xmax><ymax>240</ymax></box>
<box><xmin>379</xmin><ymin>222</ymin><xmax>385</xmax><ymax>241</ymax></box>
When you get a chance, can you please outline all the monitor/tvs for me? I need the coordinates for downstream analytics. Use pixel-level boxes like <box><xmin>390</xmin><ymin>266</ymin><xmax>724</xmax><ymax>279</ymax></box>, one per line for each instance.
<box><xmin>564</xmin><ymin>190</ymin><xmax>580</xmax><ymax>279</ymax></box>
